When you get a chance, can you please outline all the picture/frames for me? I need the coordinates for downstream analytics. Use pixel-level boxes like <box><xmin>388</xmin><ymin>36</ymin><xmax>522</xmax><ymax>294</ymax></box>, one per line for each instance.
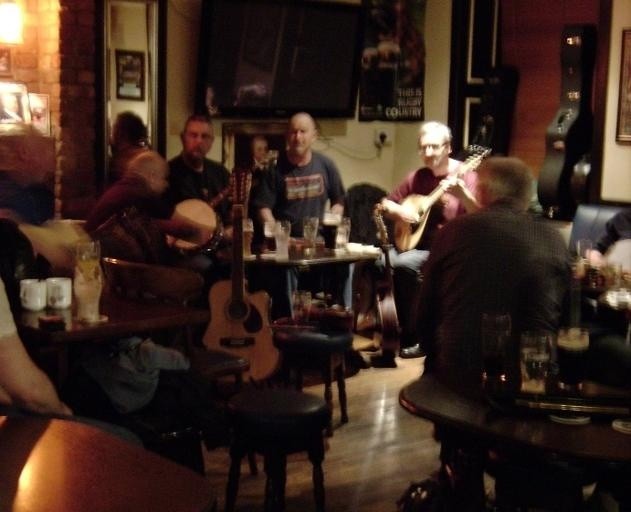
<box><xmin>0</xmin><ymin>48</ymin><xmax>13</xmax><ymax>77</ymax></box>
<box><xmin>616</xmin><ymin>28</ymin><xmax>631</xmax><ymax>145</ymax></box>
<box><xmin>115</xmin><ymin>48</ymin><xmax>145</xmax><ymax>102</ymax></box>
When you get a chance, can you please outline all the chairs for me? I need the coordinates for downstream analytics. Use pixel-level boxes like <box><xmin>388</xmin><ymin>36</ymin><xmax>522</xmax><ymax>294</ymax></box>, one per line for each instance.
<box><xmin>103</xmin><ymin>257</ymin><xmax>204</xmax><ymax>307</ymax></box>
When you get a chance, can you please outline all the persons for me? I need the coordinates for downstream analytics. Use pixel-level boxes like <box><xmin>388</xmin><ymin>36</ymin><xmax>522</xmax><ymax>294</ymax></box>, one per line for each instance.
<box><xmin>473</xmin><ymin>113</ymin><xmax>504</xmax><ymax>156</ymax></box>
<box><xmin>594</xmin><ymin>204</ymin><xmax>631</xmax><ymax>247</ymax></box>
<box><xmin>109</xmin><ymin>112</ymin><xmax>150</xmax><ymax>190</ymax></box>
<box><xmin>250</xmin><ymin>113</ymin><xmax>345</xmax><ymax>255</ymax></box>
<box><xmin>383</xmin><ymin>121</ymin><xmax>482</xmax><ymax>358</ymax></box>
<box><xmin>0</xmin><ymin>279</ymin><xmax>144</xmax><ymax>416</ymax></box>
<box><xmin>0</xmin><ymin>171</ymin><xmax>91</xmax><ymax>276</ymax></box>
<box><xmin>165</xmin><ymin>116</ymin><xmax>230</xmax><ymax>229</ymax></box>
<box><xmin>227</xmin><ymin>135</ymin><xmax>275</xmax><ymax>228</ymax></box>
<box><xmin>418</xmin><ymin>158</ymin><xmax>578</xmax><ymax>498</ymax></box>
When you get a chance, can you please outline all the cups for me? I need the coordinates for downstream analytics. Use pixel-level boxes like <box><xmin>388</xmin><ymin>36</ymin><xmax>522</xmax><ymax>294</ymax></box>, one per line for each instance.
<box><xmin>480</xmin><ymin>310</ymin><xmax>590</xmax><ymax>395</ymax></box>
<box><xmin>604</xmin><ymin>263</ymin><xmax>623</xmax><ymax>288</ymax></box>
<box><xmin>288</xmin><ymin>289</ymin><xmax>312</xmax><ymax>325</ymax></box>
<box><xmin>19</xmin><ymin>240</ymin><xmax>104</xmax><ymax>322</ymax></box>
<box><xmin>243</xmin><ymin>216</ymin><xmax>350</xmax><ymax>258</ymax></box>
<box><xmin>575</xmin><ymin>238</ymin><xmax>593</xmax><ymax>271</ymax></box>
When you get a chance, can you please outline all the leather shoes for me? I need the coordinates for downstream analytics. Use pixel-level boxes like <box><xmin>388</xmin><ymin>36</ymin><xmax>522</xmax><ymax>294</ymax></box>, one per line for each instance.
<box><xmin>401</xmin><ymin>344</ymin><xmax>424</xmax><ymax>358</ymax></box>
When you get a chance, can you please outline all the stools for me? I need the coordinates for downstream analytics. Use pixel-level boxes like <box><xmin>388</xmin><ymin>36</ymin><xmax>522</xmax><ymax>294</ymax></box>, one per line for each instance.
<box><xmin>224</xmin><ymin>388</ymin><xmax>327</xmax><ymax>512</ymax></box>
<box><xmin>180</xmin><ymin>350</ymin><xmax>250</xmax><ymax>453</ymax></box>
<box><xmin>276</xmin><ymin>313</ymin><xmax>352</xmax><ymax>437</ymax></box>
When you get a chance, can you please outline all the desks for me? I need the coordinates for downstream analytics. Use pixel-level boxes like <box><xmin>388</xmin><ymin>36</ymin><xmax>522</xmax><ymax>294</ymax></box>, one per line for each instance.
<box><xmin>247</xmin><ymin>248</ymin><xmax>383</xmax><ymax>361</ymax></box>
<box><xmin>0</xmin><ymin>416</ymin><xmax>216</xmax><ymax>511</ymax></box>
<box><xmin>398</xmin><ymin>371</ymin><xmax>630</xmax><ymax>480</ymax></box>
<box><xmin>16</xmin><ymin>300</ymin><xmax>210</xmax><ymax>344</ymax></box>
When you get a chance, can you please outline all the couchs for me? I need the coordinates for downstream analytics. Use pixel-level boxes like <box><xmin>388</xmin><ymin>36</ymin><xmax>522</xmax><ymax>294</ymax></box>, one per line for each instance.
<box><xmin>568</xmin><ymin>200</ymin><xmax>631</xmax><ymax>255</ymax></box>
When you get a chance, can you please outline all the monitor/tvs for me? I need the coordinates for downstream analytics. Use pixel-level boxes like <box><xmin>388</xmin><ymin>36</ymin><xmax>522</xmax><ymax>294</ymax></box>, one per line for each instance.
<box><xmin>196</xmin><ymin>0</ymin><xmax>368</xmax><ymax>118</ymax></box>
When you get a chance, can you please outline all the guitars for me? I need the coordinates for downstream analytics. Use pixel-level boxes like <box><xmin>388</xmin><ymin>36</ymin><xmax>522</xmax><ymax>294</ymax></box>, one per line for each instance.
<box><xmin>202</xmin><ymin>168</ymin><xmax>279</xmax><ymax>387</ymax></box>
